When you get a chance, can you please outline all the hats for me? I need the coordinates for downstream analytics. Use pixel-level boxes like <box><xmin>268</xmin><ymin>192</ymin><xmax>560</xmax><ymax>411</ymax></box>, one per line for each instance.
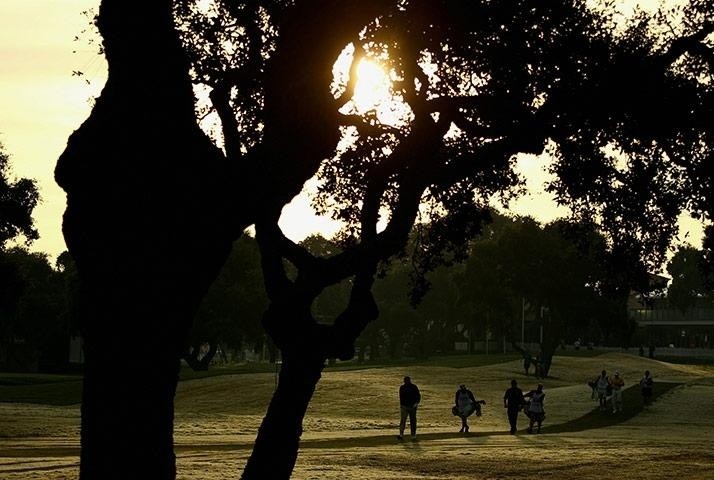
<box><xmin>613</xmin><ymin>370</ymin><xmax>620</xmax><ymax>375</ymax></box>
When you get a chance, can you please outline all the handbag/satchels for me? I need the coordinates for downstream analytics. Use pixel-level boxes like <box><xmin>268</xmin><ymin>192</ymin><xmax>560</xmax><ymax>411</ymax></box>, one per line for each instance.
<box><xmin>524</xmin><ymin>400</ymin><xmax>545</xmax><ymax>421</ymax></box>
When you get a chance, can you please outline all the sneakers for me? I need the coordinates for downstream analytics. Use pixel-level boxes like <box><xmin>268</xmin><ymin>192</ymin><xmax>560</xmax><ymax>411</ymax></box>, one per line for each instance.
<box><xmin>537</xmin><ymin>430</ymin><xmax>543</xmax><ymax>434</ymax></box>
<box><xmin>510</xmin><ymin>427</ymin><xmax>517</xmax><ymax>435</ymax></box>
<box><xmin>410</xmin><ymin>437</ymin><xmax>417</xmax><ymax>440</ymax></box>
<box><xmin>527</xmin><ymin>429</ymin><xmax>534</xmax><ymax>433</ymax></box>
<box><xmin>459</xmin><ymin>426</ymin><xmax>469</xmax><ymax>433</ymax></box>
<box><xmin>612</xmin><ymin>408</ymin><xmax>624</xmax><ymax>413</ymax></box>
<box><xmin>600</xmin><ymin>407</ymin><xmax>606</xmax><ymax>411</ymax></box>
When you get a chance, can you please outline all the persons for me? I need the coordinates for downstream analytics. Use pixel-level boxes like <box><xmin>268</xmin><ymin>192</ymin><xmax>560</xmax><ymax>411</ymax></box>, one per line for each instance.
<box><xmin>522</xmin><ymin>354</ymin><xmax>530</xmax><ymax>375</ymax></box>
<box><xmin>639</xmin><ymin>370</ymin><xmax>654</xmax><ymax>400</ymax></box>
<box><xmin>450</xmin><ymin>384</ymin><xmax>477</xmax><ymax>434</ymax></box>
<box><xmin>502</xmin><ymin>379</ymin><xmax>523</xmax><ymax>435</ymax></box>
<box><xmin>523</xmin><ymin>383</ymin><xmax>545</xmax><ymax>434</ymax></box>
<box><xmin>609</xmin><ymin>370</ymin><xmax>624</xmax><ymax>413</ymax></box>
<box><xmin>393</xmin><ymin>376</ymin><xmax>420</xmax><ymax>440</ymax></box>
<box><xmin>574</xmin><ymin>339</ymin><xmax>580</xmax><ymax>350</ymax></box>
<box><xmin>594</xmin><ymin>370</ymin><xmax>611</xmax><ymax>412</ymax></box>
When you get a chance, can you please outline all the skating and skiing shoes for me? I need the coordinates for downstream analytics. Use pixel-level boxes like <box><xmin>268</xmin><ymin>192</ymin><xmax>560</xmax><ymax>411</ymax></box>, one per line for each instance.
<box><xmin>396</xmin><ymin>435</ymin><xmax>404</xmax><ymax>441</ymax></box>
